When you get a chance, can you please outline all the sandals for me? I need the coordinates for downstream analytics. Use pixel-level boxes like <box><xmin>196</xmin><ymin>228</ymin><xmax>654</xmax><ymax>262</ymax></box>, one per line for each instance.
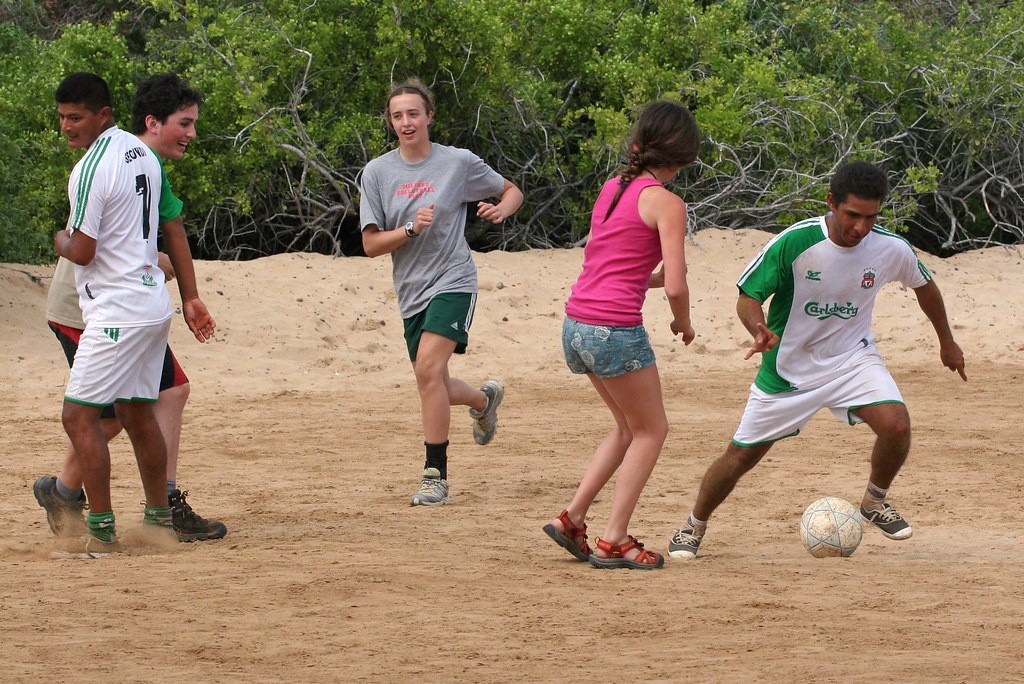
<box><xmin>589</xmin><ymin>535</ymin><xmax>664</xmax><ymax>570</ymax></box>
<box><xmin>542</xmin><ymin>510</ymin><xmax>594</xmax><ymax>562</ymax></box>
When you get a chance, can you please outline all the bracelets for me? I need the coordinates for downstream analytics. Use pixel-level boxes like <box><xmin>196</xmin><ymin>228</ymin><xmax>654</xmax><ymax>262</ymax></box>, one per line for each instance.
<box><xmin>404</xmin><ymin>228</ymin><xmax>414</xmax><ymax>238</ymax></box>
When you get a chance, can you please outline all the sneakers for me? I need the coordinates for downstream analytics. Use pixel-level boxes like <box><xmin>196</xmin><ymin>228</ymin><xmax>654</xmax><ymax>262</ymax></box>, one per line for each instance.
<box><xmin>167</xmin><ymin>489</ymin><xmax>227</xmax><ymax>542</ymax></box>
<box><xmin>667</xmin><ymin>516</ymin><xmax>706</xmax><ymax>561</ymax></box>
<box><xmin>33</xmin><ymin>475</ymin><xmax>90</xmax><ymax>537</ymax></box>
<box><xmin>858</xmin><ymin>502</ymin><xmax>913</xmax><ymax>540</ymax></box>
<box><xmin>410</xmin><ymin>468</ymin><xmax>449</xmax><ymax>506</ymax></box>
<box><xmin>47</xmin><ymin>535</ymin><xmax>123</xmax><ymax>559</ymax></box>
<box><xmin>468</xmin><ymin>379</ymin><xmax>503</xmax><ymax>445</ymax></box>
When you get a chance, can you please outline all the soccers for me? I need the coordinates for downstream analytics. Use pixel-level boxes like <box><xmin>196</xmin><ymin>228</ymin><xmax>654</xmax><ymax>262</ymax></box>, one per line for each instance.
<box><xmin>799</xmin><ymin>496</ymin><xmax>864</xmax><ymax>558</ymax></box>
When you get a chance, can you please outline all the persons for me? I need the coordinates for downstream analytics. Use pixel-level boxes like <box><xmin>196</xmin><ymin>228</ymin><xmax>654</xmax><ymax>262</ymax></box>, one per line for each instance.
<box><xmin>540</xmin><ymin>100</ymin><xmax>699</xmax><ymax>570</ymax></box>
<box><xmin>31</xmin><ymin>69</ymin><xmax>226</xmax><ymax>559</ymax></box>
<box><xmin>667</xmin><ymin>162</ymin><xmax>968</xmax><ymax>557</ymax></box>
<box><xmin>360</xmin><ymin>80</ymin><xmax>522</xmax><ymax>506</ymax></box>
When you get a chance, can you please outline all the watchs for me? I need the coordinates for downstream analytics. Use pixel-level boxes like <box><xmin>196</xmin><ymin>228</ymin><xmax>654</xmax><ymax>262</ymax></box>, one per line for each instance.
<box><xmin>406</xmin><ymin>220</ymin><xmax>420</xmax><ymax>236</ymax></box>
